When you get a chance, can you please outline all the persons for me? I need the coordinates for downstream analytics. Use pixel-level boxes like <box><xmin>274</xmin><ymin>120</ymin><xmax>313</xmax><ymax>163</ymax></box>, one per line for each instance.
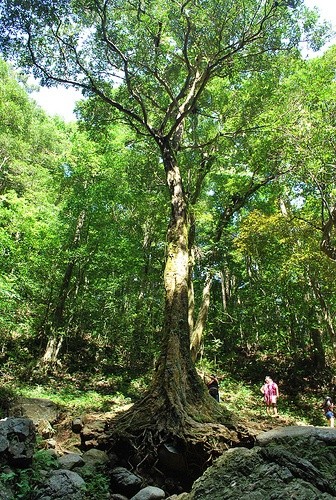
<box><xmin>204</xmin><ymin>372</ymin><xmax>219</xmax><ymax>404</ymax></box>
<box><xmin>260</xmin><ymin>376</ymin><xmax>280</xmax><ymax>419</ymax></box>
<box><xmin>323</xmin><ymin>396</ymin><xmax>336</xmax><ymax>428</ymax></box>
